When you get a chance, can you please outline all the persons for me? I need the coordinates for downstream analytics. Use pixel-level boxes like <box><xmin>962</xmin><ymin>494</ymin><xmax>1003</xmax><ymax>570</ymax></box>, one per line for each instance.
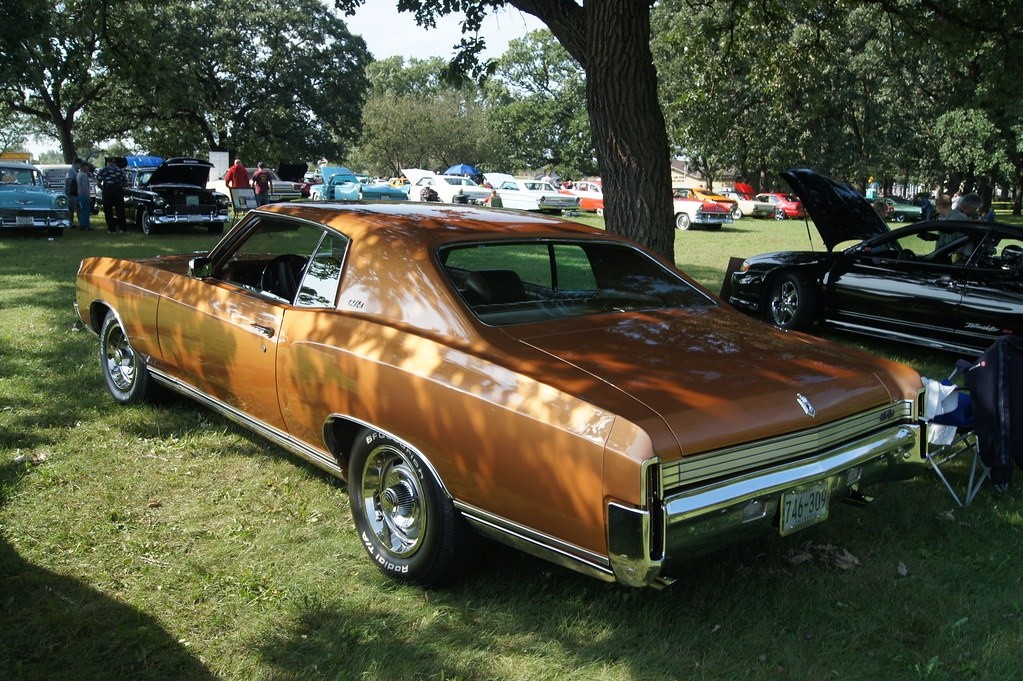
<box><xmin>64</xmin><ymin>157</ymin><xmax>83</xmax><ymax>228</ymax></box>
<box><xmin>75</xmin><ymin>160</ymin><xmax>94</xmax><ymax>231</ymax></box>
<box><xmin>914</xmin><ymin>194</ymin><xmax>933</xmax><ymax>222</ymax></box>
<box><xmin>418</xmin><ymin>179</ymin><xmax>503</xmax><ymax>207</ymax></box>
<box><xmin>248</xmin><ymin>161</ymin><xmax>273</xmax><ymax>209</ymax></box>
<box><xmin>94</xmin><ymin>155</ymin><xmax>130</xmax><ymax>234</ymax></box>
<box><xmin>873</xmin><ymin>197</ymin><xmax>896</xmax><ymax>223</ymax></box>
<box><xmin>933</xmin><ymin>188</ymin><xmax>995</xmax><ymax>267</ymax></box>
<box><xmin>224</xmin><ymin>159</ymin><xmax>250</xmax><ymax>193</ymax></box>
<box><xmin>565</xmin><ymin>180</ymin><xmax>578</xmax><ymax>190</ymax></box>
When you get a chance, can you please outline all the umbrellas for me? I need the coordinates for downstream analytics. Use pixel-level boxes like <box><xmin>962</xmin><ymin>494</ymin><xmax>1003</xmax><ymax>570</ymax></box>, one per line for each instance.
<box><xmin>443</xmin><ymin>162</ymin><xmax>479</xmax><ymax>180</ymax></box>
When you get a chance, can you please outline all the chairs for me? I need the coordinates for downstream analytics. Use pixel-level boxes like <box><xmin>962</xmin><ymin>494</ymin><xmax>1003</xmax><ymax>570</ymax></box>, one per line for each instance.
<box><xmin>458</xmin><ymin>271</ymin><xmax>527</xmax><ymax>304</ymax></box>
<box><xmin>925</xmin><ymin>360</ymin><xmax>1008</xmax><ymax>506</ymax></box>
<box><xmin>986</xmin><ymin>245</ymin><xmax>1022</xmax><ymax>271</ymax></box>
<box><xmin>954</xmin><ymin>241</ymin><xmax>984</xmax><ymax>267</ymax></box>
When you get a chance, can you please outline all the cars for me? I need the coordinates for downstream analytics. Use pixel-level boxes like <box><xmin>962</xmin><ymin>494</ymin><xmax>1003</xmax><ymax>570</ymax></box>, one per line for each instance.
<box><xmin>308</xmin><ymin>166</ymin><xmax>408</xmax><ymax>202</ymax></box>
<box><xmin>674</xmin><ymin>188</ymin><xmax>738</xmax><ymax>213</ymax></box>
<box><xmin>729</xmin><ymin>170</ymin><xmax>1023</xmax><ymax>362</ymax></box>
<box><xmin>559</xmin><ymin>180</ymin><xmax>604</xmax><ymax>213</ymax></box>
<box><xmin>671</xmin><ymin>196</ymin><xmax>734</xmax><ymax>229</ymax></box>
<box><xmin>36</xmin><ymin>165</ymin><xmax>103</xmax><ymax>216</ymax></box>
<box><xmin>72</xmin><ymin>201</ymin><xmax>926</xmax><ymax>600</ymax></box>
<box><xmin>205</xmin><ymin>167</ymin><xmax>302</xmax><ymax>205</ymax></box>
<box><xmin>96</xmin><ymin>156</ymin><xmax>231</xmax><ymax>236</ymax></box>
<box><xmin>483</xmin><ymin>172</ymin><xmax>581</xmax><ymax>214</ymax></box>
<box><xmin>0</xmin><ymin>158</ymin><xmax>70</xmax><ymax>237</ymax></box>
<box><xmin>863</xmin><ymin>193</ymin><xmax>926</xmax><ymax>223</ymax></box>
<box><xmin>735</xmin><ymin>181</ymin><xmax>808</xmax><ymax>218</ymax></box>
<box><xmin>717</xmin><ymin>191</ymin><xmax>776</xmax><ymax>221</ymax></box>
<box><xmin>399</xmin><ymin>168</ymin><xmax>493</xmax><ymax>206</ymax></box>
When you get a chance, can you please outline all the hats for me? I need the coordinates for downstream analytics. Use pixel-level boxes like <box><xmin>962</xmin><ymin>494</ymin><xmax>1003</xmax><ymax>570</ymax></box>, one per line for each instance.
<box><xmin>73</xmin><ymin>158</ymin><xmax>83</xmax><ymax>163</ymax></box>
<box><xmin>80</xmin><ymin>162</ymin><xmax>91</xmax><ymax>167</ymax></box>
<box><xmin>108</xmin><ymin>158</ymin><xmax>117</xmax><ymax>163</ymax></box>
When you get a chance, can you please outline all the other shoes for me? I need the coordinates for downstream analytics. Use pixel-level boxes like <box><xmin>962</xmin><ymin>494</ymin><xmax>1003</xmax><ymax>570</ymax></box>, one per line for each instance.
<box><xmin>107</xmin><ymin>231</ymin><xmax>117</xmax><ymax>235</ymax></box>
<box><xmin>88</xmin><ymin>228</ymin><xmax>93</xmax><ymax>231</ymax></box>
<box><xmin>69</xmin><ymin>225</ymin><xmax>76</xmax><ymax>229</ymax></box>
<box><xmin>80</xmin><ymin>227</ymin><xmax>86</xmax><ymax>230</ymax></box>
<box><xmin>118</xmin><ymin>230</ymin><xmax>129</xmax><ymax>234</ymax></box>
